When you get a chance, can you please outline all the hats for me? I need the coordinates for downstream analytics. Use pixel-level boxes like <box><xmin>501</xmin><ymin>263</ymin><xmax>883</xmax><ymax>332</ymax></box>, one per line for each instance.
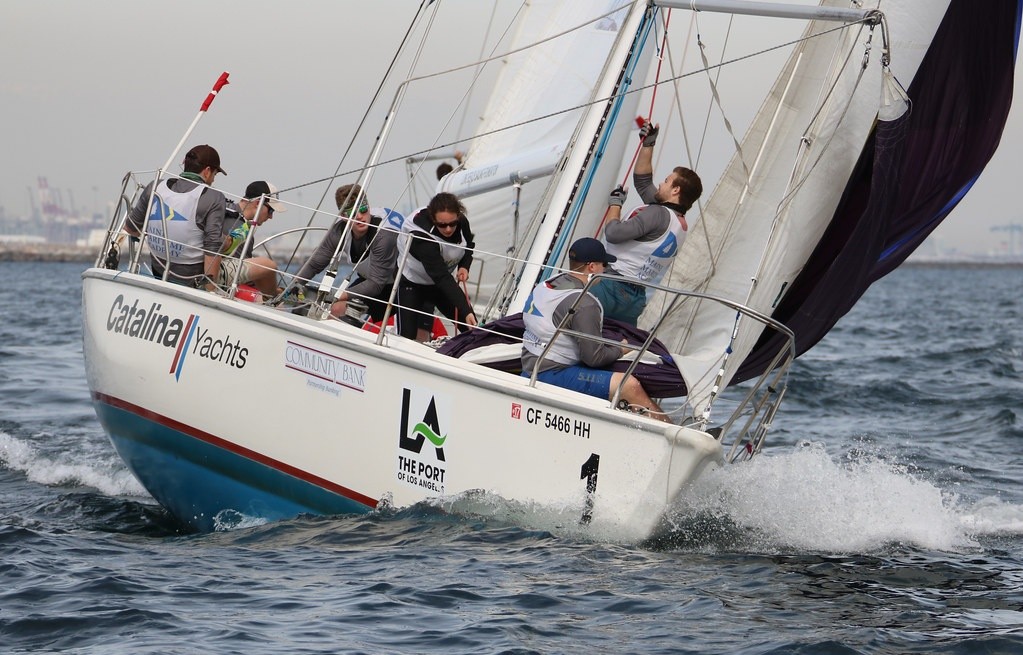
<box><xmin>569</xmin><ymin>238</ymin><xmax>617</xmax><ymax>263</ymax></box>
<box><xmin>186</xmin><ymin>144</ymin><xmax>227</xmax><ymax>175</ymax></box>
<box><xmin>245</xmin><ymin>181</ymin><xmax>288</xmax><ymax>212</ymax></box>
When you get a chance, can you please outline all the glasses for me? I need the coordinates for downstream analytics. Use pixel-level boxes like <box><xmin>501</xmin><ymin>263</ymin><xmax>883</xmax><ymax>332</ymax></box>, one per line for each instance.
<box><xmin>434</xmin><ymin>219</ymin><xmax>459</xmax><ymax>228</ymax></box>
<box><xmin>264</xmin><ymin>204</ymin><xmax>275</xmax><ymax>215</ymax></box>
<box><xmin>343</xmin><ymin>205</ymin><xmax>368</xmax><ymax>217</ymax></box>
<box><xmin>602</xmin><ymin>261</ymin><xmax>608</xmax><ymax>267</ymax></box>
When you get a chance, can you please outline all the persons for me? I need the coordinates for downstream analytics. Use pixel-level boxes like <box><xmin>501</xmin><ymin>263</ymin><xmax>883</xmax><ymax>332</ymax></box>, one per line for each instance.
<box><xmin>124</xmin><ymin>144</ymin><xmax>287</xmax><ymax>300</ymax></box>
<box><xmin>293</xmin><ymin>183</ymin><xmax>406</xmax><ymax>327</ymax></box>
<box><xmin>393</xmin><ymin>192</ymin><xmax>478</xmax><ymax>340</ymax></box>
<box><xmin>520</xmin><ymin>236</ymin><xmax>675</xmax><ymax>424</ymax></box>
<box><xmin>222</xmin><ymin>180</ymin><xmax>287</xmax><ymax>260</ymax></box>
<box><xmin>435</xmin><ymin>150</ymin><xmax>462</xmax><ymax>181</ymax></box>
<box><xmin>588</xmin><ymin>118</ymin><xmax>703</xmax><ymax>327</ymax></box>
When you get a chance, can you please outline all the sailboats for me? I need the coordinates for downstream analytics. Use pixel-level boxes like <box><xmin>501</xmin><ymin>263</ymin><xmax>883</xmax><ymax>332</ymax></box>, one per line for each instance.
<box><xmin>77</xmin><ymin>0</ymin><xmax>1020</xmax><ymax>549</ymax></box>
